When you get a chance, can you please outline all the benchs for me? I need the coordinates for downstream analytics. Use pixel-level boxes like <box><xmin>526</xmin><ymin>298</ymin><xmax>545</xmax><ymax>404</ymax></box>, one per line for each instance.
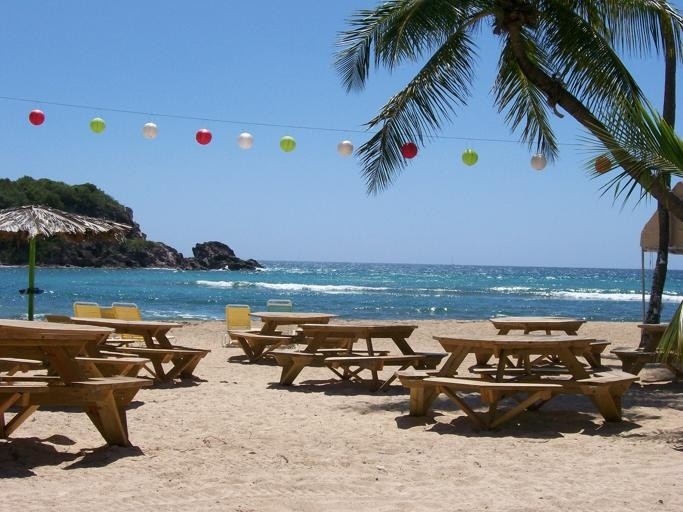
<box><xmin>611</xmin><ymin>347</ymin><xmax>682</xmax><ymax>383</ymax></box>
<box><xmin>107</xmin><ymin>338</ymin><xmax>212</xmax><ymax>383</ymax></box>
<box><xmin>267</xmin><ymin>348</ymin><xmax>390</xmax><ymax>385</ymax></box>
<box><xmin>397</xmin><ymin>367</ymin><xmax>562</xmax><ymax>436</ymax></box>
<box><xmin>228</xmin><ymin>327</ymin><xmax>302</xmax><ymax>362</ymax></box>
<box><xmin>583</xmin><ymin>339</ymin><xmax>611</xmax><ymax>367</ymax></box>
<box><xmin>324</xmin><ymin>353</ymin><xmax>448</xmax><ymax>391</ymax></box>
<box><xmin>578</xmin><ymin>372</ymin><xmax>640</xmax><ymax>423</ymax></box>
<box><xmin>0</xmin><ymin>351</ymin><xmax>153</xmax><ymax>446</ymax></box>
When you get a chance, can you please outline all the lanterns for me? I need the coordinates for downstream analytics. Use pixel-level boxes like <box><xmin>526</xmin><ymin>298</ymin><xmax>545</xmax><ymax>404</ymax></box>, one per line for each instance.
<box><xmin>196</xmin><ymin>128</ymin><xmax>212</xmax><ymax>145</ymax></box>
<box><xmin>336</xmin><ymin>140</ymin><xmax>353</xmax><ymax>158</ymax></box>
<box><xmin>280</xmin><ymin>136</ymin><xmax>296</xmax><ymax>152</ymax></box>
<box><xmin>28</xmin><ymin>109</ymin><xmax>45</xmax><ymax>125</ymax></box>
<box><xmin>142</xmin><ymin>122</ymin><xmax>159</xmax><ymax>138</ymax></box>
<box><xmin>401</xmin><ymin>143</ymin><xmax>417</xmax><ymax>158</ymax></box>
<box><xmin>90</xmin><ymin>118</ymin><xmax>106</xmax><ymax>133</ymax></box>
<box><xmin>462</xmin><ymin>149</ymin><xmax>478</xmax><ymax>165</ymax></box>
<box><xmin>531</xmin><ymin>154</ymin><xmax>546</xmax><ymax>170</ymax></box>
<box><xmin>238</xmin><ymin>133</ymin><xmax>253</xmax><ymax>148</ymax></box>
<box><xmin>595</xmin><ymin>156</ymin><xmax>611</xmax><ymax>173</ymax></box>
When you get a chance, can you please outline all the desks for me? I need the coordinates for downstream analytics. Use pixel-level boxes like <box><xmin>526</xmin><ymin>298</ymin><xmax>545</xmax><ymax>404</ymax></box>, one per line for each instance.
<box><xmin>488</xmin><ymin>316</ymin><xmax>586</xmax><ymax>335</ymax></box>
<box><xmin>637</xmin><ymin>324</ymin><xmax>670</xmax><ymax>346</ymax></box>
<box><xmin>70</xmin><ymin>316</ymin><xmax>183</xmax><ymax>346</ymax></box>
<box><xmin>296</xmin><ymin>323</ymin><xmax>418</xmax><ymax>354</ymax></box>
<box><xmin>0</xmin><ymin>319</ymin><xmax>116</xmax><ymax>349</ymax></box>
<box><xmin>432</xmin><ymin>334</ymin><xmax>597</xmax><ymax>380</ymax></box>
<box><xmin>248</xmin><ymin>311</ymin><xmax>340</xmax><ymax>335</ymax></box>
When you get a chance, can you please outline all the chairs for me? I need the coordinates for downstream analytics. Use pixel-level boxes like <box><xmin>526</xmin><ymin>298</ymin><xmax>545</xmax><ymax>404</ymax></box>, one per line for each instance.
<box><xmin>222</xmin><ymin>304</ymin><xmax>252</xmax><ymax>350</ymax></box>
<box><xmin>111</xmin><ymin>301</ymin><xmax>175</xmax><ymax>345</ymax></box>
<box><xmin>74</xmin><ymin>300</ymin><xmax>103</xmax><ymax>320</ymax></box>
